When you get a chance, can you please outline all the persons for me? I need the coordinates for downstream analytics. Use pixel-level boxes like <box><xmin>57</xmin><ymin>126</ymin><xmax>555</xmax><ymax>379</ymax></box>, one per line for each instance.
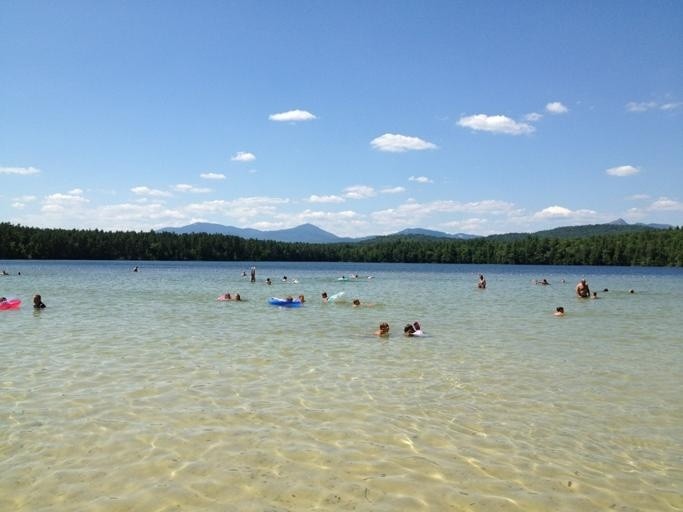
<box><xmin>342</xmin><ymin>273</ymin><xmax>358</xmax><ymax>279</ymax></box>
<box><xmin>536</xmin><ymin>279</ymin><xmax>634</xmax><ymax>317</ymax></box>
<box><xmin>373</xmin><ymin>321</ymin><xmax>423</xmax><ymax>337</ymax></box>
<box><xmin>133</xmin><ymin>266</ymin><xmax>138</xmax><ymax>272</ymax></box>
<box><xmin>478</xmin><ymin>275</ymin><xmax>485</xmax><ymax>288</ymax></box>
<box><xmin>322</xmin><ymin>291</ymin><xmax>374</xmax><ymax>308</ymax></box>
<box><xmin>218</xmin><ymin>267</ymin><xmax>304</xmax><ymax>303</ymax></box>
<box><xmin>0</xmin><ymin>294</ymin><xmax>45</xmax><ymax>310</ymax></box>
<box><xmin>0</xmin><ymin>270</ymin><xmax>21</xmax><ymax>276</ymax></box>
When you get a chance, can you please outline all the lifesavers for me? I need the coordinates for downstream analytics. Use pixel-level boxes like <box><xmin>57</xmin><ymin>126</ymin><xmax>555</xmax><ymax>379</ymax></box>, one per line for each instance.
<box><xmin>1</xmin><ymin>297</ymin><xmax>22</xmax><ymax>310</ymax></box>
<box><xmin>267</xmin><ymin>296</ymin><xmax>302</xmax><ymax>307</ymax></box>
<box><xmin>216</xmin><ymin>294</ymin><xmax>237</xmax><ymax>301</ymax></box>
